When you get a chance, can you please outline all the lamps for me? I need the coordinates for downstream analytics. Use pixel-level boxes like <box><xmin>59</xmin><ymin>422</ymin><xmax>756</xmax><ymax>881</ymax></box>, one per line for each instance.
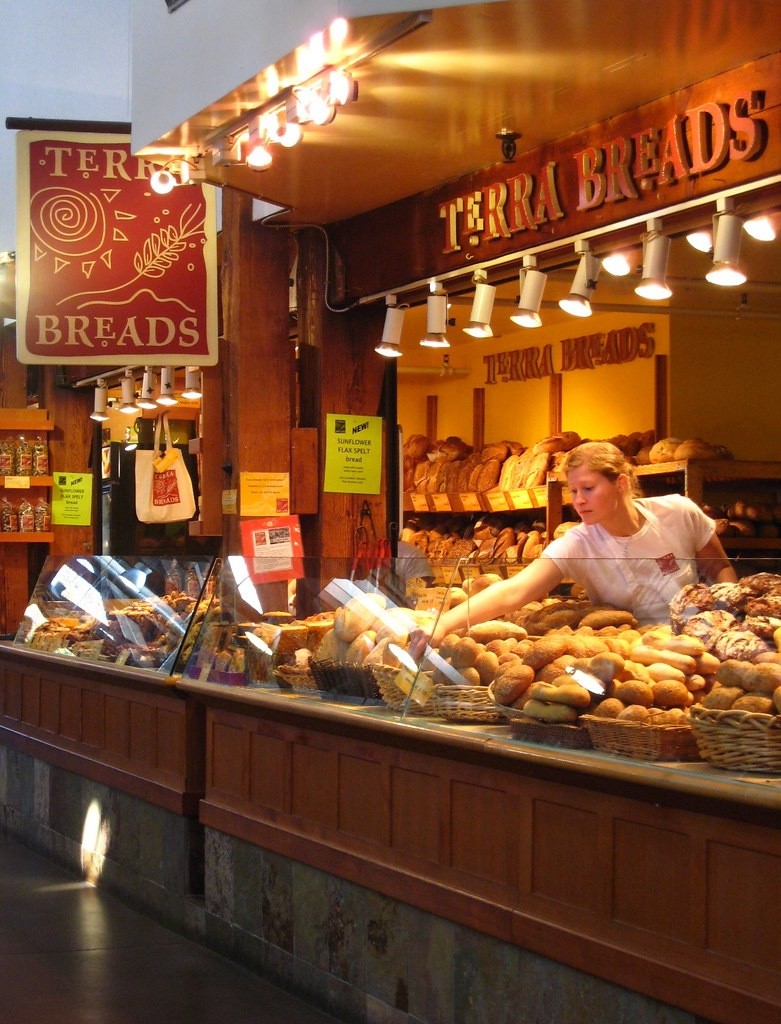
<box><xmin>138</xmin><ymin>366</ymin><xmax>157</xmax><ymax>410</ymax></box>
<box><xmin>704</xmin><ymin>199</ymin><xmax>746</xmax><ymax>287</ymax></box>
<box><xmin>181</xmin><ymin>367</ymin><xmax>202</xmax><ymax>400</ymax></box>
<box><xmin>420</xmin><ymin>282</ymin><xmax>451</xmax><ymax>348</ymax></box>
<box><xmin>510</xmin><ymin>255</ymin><xmax>547</xmax><ymax>328</ymax></box>
<box><xmin>118</xmin><ymin>370</ymin><xmax>139</xmax><ymax>414</ymax></box>
<box><xmin>633</xmin><ymin>218</ymin><xmax>674</xmax><ymax>301</ymax></box>
<box><xmin>155</xmin><ymin>366</ymin><xmax>179</xmax><ymax>405</ymax></box>
<box><xmin>150</xmin><ymin>12</ymin><xmax>433</xmax><ymax>194</ymax></box>
<box><xmin>557</xmin><ymin>239</ymin><xmax>602</xmax><ymax>318</ymax></box>
<box><xmin>90</xmin><ymin>379</ymin><xmax>110</xmax><ymax>421</ymax></box>
<box><xmin>375</xmin><ymin>294</ymin><xmax>403</xmax><ymax>357</ymax></box>
<box><xmin>461</xmin><ymin>268</ymin><xmax>495</xmax><ymax>339</ymax></box>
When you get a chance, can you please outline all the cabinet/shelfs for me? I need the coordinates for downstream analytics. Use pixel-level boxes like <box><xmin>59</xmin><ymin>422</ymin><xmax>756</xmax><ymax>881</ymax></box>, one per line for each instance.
<box><xmin>0</xmin><ymin>408</ymin><xmax>57</xmax><ymax>542</ymax></box>
<box><xmin>401</xmin><ymin>459</ymin><xmax>781</xmax><ymax>611</ymax></box>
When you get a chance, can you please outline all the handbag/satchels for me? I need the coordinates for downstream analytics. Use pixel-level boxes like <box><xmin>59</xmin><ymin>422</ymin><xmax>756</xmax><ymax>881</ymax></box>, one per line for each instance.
<box><xmin>135</xmin><ymin>410</ymin><xmax>196</xmax><ymax>524</ymax></box>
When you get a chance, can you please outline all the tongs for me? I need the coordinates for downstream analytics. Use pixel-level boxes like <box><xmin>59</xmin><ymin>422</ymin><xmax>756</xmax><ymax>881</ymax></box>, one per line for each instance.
<box><xmin>350</xmin><ymin>538</ymin><xmax>393</xmax><ymax>593</ymax></box>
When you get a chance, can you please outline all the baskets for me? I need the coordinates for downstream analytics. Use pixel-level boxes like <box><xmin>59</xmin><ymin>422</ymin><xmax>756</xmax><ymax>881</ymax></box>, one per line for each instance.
<box><xmin>271</xmin><ymin>655</ymin><xmax>781</xmax><ymax>774</ymax></box>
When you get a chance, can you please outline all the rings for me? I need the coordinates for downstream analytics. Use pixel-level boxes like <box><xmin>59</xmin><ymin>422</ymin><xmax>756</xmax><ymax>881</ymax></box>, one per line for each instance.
<box><xmin>408</xmin><ymin>641</ymin><xmax>416</xmax><ymax>648</ymax></box>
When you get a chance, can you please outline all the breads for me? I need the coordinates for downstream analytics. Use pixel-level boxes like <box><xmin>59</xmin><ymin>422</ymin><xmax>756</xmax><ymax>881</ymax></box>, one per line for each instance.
<box><xmin>399</xmin><ymin>430</ymin><xmax>781</xmax><ymax>566</ymax></box>
<box><xmin>27</xmin><ymin>590</ymin><xmax>336</xmax><ymax>680</ymax></box>
<box><xmin>309</xmin><ymin>573</ymin><xmax>781</xmax><ymax>727</ymax></box>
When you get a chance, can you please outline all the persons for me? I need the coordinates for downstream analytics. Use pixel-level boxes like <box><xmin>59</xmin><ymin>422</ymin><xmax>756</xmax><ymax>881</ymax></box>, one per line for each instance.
<box><xmin>407</xmin><ymin>441</ymin><xmax>742</xmax><ymax>662</ymax></box>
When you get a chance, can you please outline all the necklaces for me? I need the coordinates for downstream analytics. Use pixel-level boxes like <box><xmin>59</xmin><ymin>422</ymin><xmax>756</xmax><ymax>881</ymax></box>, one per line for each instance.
<box><xmin>601</xmin><ymin>511</ymin><xmax>642</xmax><ymax>557</ymax></box>
